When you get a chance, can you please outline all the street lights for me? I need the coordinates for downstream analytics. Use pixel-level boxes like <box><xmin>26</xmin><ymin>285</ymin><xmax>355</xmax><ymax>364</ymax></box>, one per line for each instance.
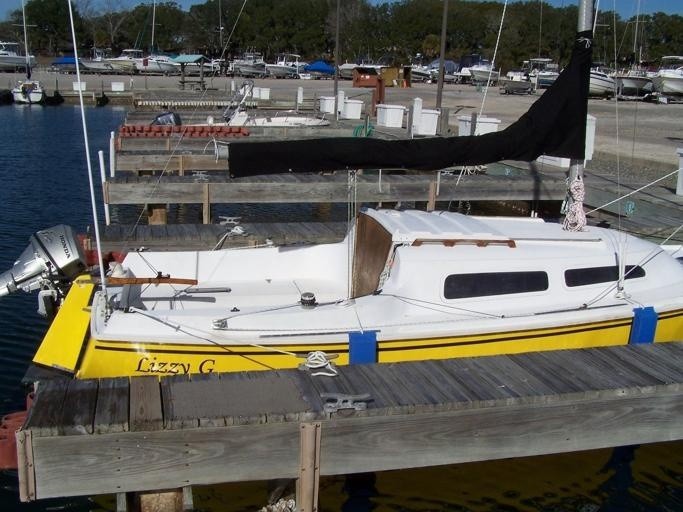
<box><xmin>220</xmin><ymin>27</ymin><xmax>224</xmax><ymax>59</ymax></box>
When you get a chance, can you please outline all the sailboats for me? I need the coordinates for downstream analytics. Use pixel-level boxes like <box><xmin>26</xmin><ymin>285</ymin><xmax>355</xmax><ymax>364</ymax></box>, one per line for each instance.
<box><xmin>0</xmin><ymin>0</ymin><xmax>682</xmax><ymax>379</ymax></box>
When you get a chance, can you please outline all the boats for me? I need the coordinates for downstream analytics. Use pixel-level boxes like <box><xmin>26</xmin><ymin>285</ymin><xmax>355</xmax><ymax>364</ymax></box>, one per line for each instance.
<box><xmin>0</xmin><ymin>50</ymin><xmax>683</xmax><ymax>103</ymax></box>
<box><xmin>183</xmin><ymin>80</ymin><xmax>330</xmax><ymax>127</ymax></box>
<box><xmin>11</xmin><ymin>80</ymin><xmax>44</xmax><ymax>103</ymax></box>
